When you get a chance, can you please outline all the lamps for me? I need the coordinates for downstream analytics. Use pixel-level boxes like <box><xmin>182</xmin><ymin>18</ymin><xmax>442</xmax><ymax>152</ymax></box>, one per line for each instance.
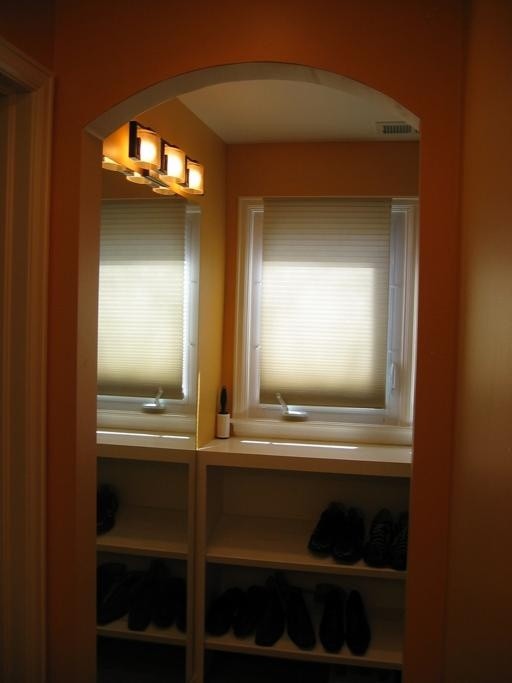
<box><xmin>102</xmin><ymin>121</ymin><xmax>205</xmax><ymax>199</ymax></box>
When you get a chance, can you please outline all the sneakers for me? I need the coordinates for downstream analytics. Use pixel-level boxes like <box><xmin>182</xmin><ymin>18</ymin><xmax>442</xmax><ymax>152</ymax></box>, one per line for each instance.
<box><xmin>97</xmin><ymin>556</ymin><xmax>186</xmax><ymax>633</ymax></box>
<box><xmin>97</xmin><ymin>484</ymin><xmax>118</xmax><ymax>536</ymax></box>
<box><xmin>204</xmin><ymin>562</ymin><xmax>370</xmax><ymax>656</ymax></box>
<box><xmin>307</xmin><ymin>502</ymin><xmax>405</xmax><ymax>570</ymax></box>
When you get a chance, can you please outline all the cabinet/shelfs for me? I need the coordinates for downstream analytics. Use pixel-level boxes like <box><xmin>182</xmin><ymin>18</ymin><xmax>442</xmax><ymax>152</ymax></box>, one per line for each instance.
<box><xmin>193</xmin><ymin>436</ymin><xmax>413</xmax><ymax>683</ymax></box>
<box><xmin>96</xmin><ymin>428</ymin><xmax>196</xmax><ymax>683</ymax></box>
